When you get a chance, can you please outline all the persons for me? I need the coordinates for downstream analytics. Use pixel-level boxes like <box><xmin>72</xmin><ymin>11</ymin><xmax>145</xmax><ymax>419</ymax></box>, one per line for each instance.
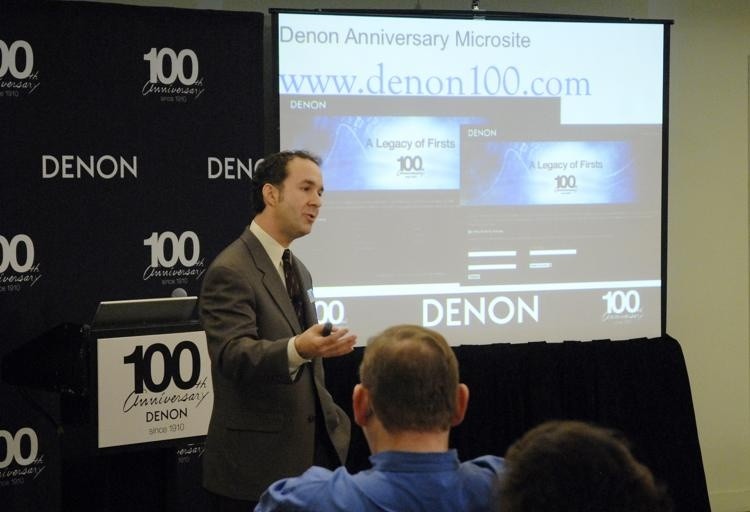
<box><xmin>255</xmin><ymin>325</ymin><xmax>508</xmax><ymax>511</ymax></box>
<box><xmin>196</xmin><ymin>149</ymin><xmax>358</xmax><ymax>511</ymax></box>
<box><xmin>487</xmin><ymin>420</ymin><xmax>656</xmax><ymax>511</ymax></box>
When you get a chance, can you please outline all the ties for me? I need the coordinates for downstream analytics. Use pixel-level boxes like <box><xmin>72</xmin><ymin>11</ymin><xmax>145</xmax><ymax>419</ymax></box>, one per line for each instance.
<box><xmin>283</xmin><ymin>249</ymin><xmax>305</xmax><ymax>329</ymax></box>
